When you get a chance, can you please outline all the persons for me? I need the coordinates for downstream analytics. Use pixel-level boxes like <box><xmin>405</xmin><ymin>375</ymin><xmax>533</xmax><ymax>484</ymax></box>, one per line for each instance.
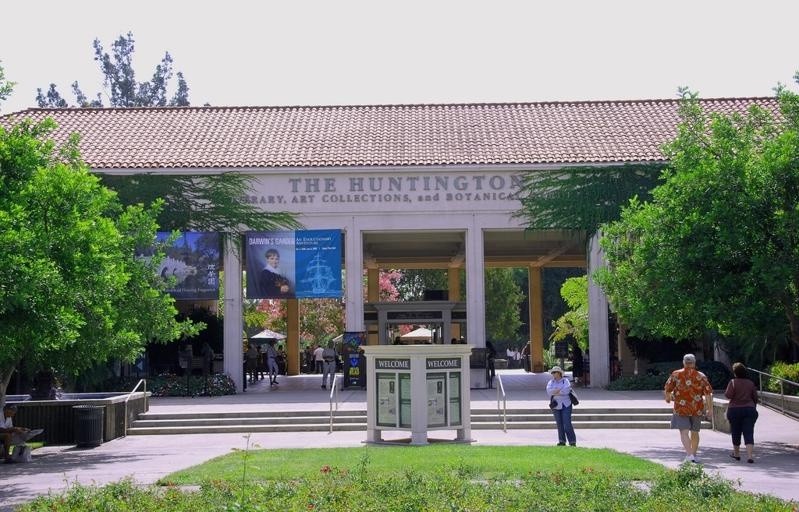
<box><xmin>258</xmin><ymin>249</ymin><xmax>293</xmax><ymax>298</ymax></box>
<box><xmin>0</xmin><ymin>402</ymin><xmax>33</xmax><ymax>464</ymax></box>
<box><xmin>723</xmin><ymin>362</ymin><xmax>760</xmax><ymax>464</ymax></box>
<box><xmin>663</xmin><ymin>350</ymin><xmax>715</xmax><ymax>465</ymax></box>
<box><xmin>244</xmin><ymin>333</ymin><xmax>527</xmax><ymax>389</ymax></box>
<box><xmin>545</xmin><ymin>364</ymin><xmax>577</xmax><ymax>447</ymax></box>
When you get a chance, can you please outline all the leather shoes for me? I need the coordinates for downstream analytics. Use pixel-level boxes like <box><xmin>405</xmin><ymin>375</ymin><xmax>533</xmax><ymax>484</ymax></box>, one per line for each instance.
<box><xmin>746</xmin><ymin>458</ymin><xmax>754</xmax><ymax>463</ymax></box>
<box><xmin>729</xmin><ymin>452</ymin><xmax>740</xmax><ymax>460</ymax></box>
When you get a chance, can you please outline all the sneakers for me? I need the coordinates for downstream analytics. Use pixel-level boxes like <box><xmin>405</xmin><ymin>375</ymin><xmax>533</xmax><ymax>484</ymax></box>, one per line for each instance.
<box><xmin>678</xmin><ymin>454</ymin><xmax>694</xmax><ymax>465</ymax></box>
<box><xmin>692</xmin><ymin>456</ymin><xmax>701</xmax><ymax>463</ymax></box>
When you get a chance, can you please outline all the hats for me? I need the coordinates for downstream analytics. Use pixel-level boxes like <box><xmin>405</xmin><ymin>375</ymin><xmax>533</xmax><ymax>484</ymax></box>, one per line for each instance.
<box><xmin>683</xmin><ymin>353</ymin><xmax>696</xmax><ymax>363</ymax></box>
<box><xmin>550</xmin><ymin>366</ymin><xmax>565</xmax><ymax>377</ymax></box>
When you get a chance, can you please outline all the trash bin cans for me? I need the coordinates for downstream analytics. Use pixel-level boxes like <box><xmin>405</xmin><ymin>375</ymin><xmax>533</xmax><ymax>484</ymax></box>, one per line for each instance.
<box><xmin>72</xmin><ymin>404</ymin><xmax>106</xmax><ymax>446</ymax></box>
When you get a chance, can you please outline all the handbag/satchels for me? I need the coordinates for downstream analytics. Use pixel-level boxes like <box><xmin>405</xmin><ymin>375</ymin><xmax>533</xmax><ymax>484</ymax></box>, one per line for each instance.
<box><xmin>568</xmin><ymin>388</ymin><xmax>579</xmax><ymax>405</ymax></box>
